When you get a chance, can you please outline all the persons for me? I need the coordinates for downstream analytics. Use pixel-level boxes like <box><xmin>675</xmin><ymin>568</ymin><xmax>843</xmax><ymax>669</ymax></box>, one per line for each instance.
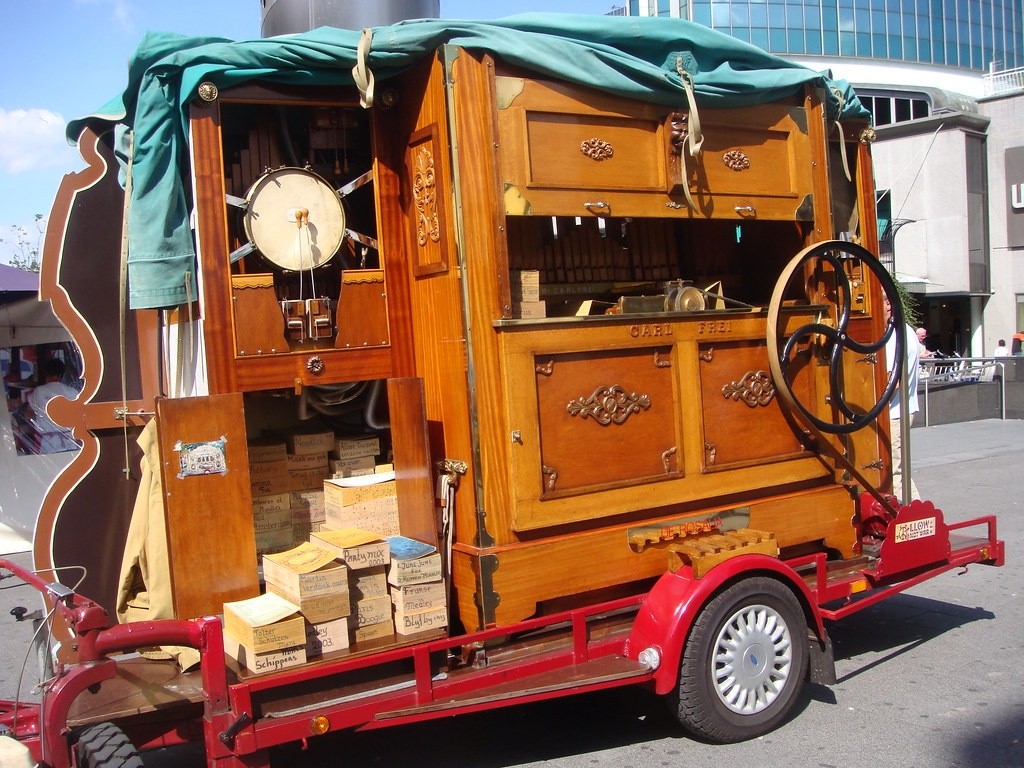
<box><xmin>884</xmin><ymin>292</ymin><xmax>922</xmax><ymax>504</ymax></box>
<box><xmin>916</xmin><ymin>328</ymin><xmax>938</xmax><ymax>379</ymax></box>
<box><xmin>994</xmin><ymin>340</ymin><xmax>1010</xmax><ymax>356</ymax></box>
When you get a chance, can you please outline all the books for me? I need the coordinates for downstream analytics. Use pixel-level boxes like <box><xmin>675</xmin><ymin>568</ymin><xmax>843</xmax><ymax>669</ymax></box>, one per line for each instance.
<box><xmin>222</xmin><ymin>108</ymin><xmax>683</xmax><ymax>674</ymax></box>
